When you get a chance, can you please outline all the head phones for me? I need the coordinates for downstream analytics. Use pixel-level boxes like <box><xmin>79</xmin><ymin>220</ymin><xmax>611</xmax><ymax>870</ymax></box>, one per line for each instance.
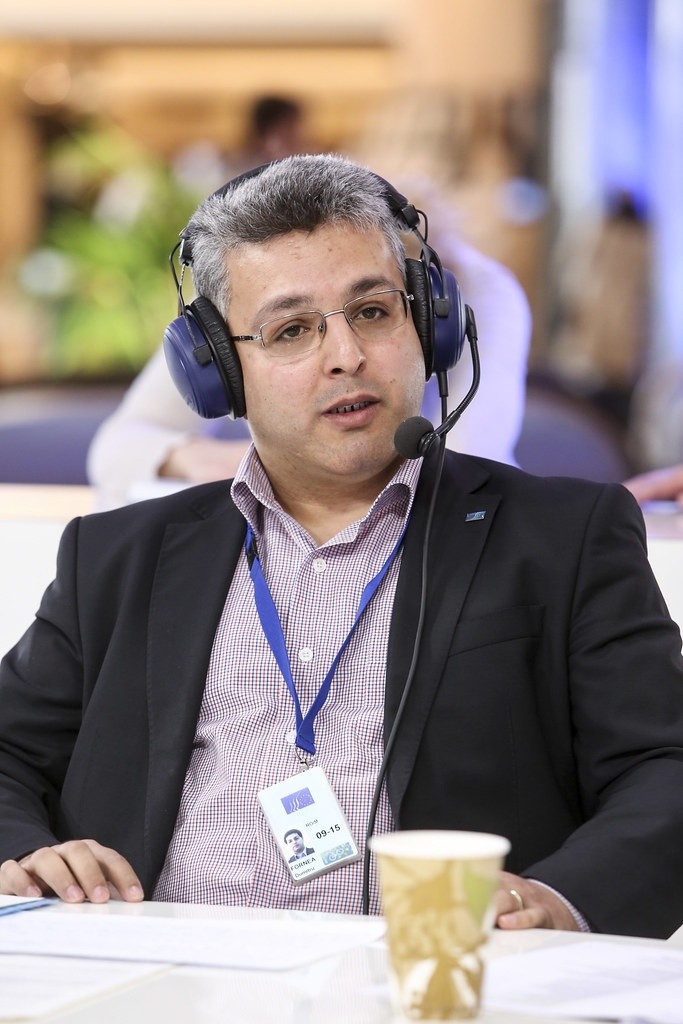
<box><xmin>164</xmin><ymin>162</ymin><xmax>477</xmax><ymax>419</ymax></box>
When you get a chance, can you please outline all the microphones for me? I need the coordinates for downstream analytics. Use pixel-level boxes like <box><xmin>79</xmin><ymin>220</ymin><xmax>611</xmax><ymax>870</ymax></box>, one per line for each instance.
<box><xmin>393</xmin><ymin>337</ymin><xmax>480</xmax><ymax>461</ymax></box>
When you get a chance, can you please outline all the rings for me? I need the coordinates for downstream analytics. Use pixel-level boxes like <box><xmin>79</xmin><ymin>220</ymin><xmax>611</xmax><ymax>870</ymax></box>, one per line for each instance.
<box><xmin>510</xmin><ymin>890</ymin><xmax>524</xmax><ymax>911</ymax></box>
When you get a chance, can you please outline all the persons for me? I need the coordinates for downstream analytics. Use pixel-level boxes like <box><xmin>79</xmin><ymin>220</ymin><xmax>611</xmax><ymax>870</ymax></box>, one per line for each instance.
<box><xmin>87</xmin><ymin>174</ymin><xmax>530</xmax><ymax>502</ymax></box>
<box><xmin>0</xmin><ymin>152</ymin><xmax>683</xmax><ymax>939</ymax></box>
<box><xmin>245</xmin><ymin>96</ymin><xmax>301</xmax><ymax>166</ymax></box>
<box><xmin>283</xmin><ymin>829</ymin><xmax>314</xmax><ymax>864</ymax></box>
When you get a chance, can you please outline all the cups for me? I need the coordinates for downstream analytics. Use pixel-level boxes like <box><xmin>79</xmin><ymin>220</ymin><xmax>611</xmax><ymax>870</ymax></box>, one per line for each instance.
<box><xmin>366</xmin><ymin>829</ymin><xmax>510</xmax><ymax>1024</ymax></box>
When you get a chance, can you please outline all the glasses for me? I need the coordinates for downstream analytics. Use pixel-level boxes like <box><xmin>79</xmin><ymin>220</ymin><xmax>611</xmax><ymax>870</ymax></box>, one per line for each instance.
<box><xmin>230</xmin><ymin>288</ymin><xmax>414</xmax><ymax>356</ymax></box>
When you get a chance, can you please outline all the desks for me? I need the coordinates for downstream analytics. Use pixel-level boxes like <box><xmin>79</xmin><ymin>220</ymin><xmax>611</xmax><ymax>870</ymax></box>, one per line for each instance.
<box><xmin>1</xmin><ymin>892</ymin><xmax>683</xmax><ymax>1024</ymax></box>
<box><xmin>2</xmin><ymin>478</ymin><xmax>683</xmax><ymax>667</ymax></box>
